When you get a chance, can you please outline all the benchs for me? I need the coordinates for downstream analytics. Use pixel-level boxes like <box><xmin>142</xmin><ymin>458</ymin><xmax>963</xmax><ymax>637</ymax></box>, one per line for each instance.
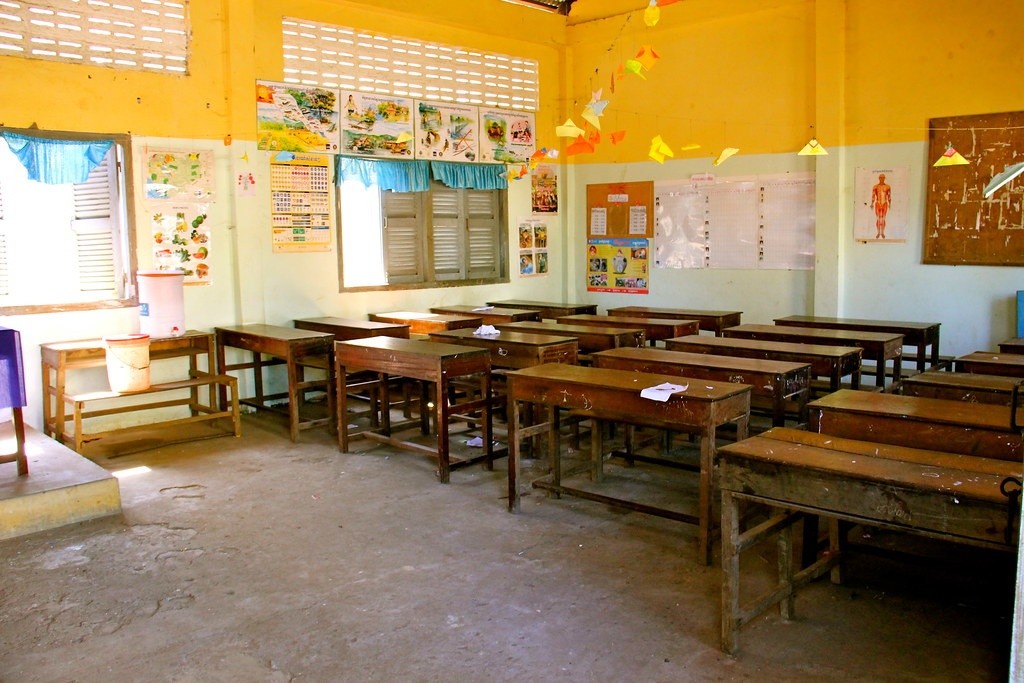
<box><xmin>62</xmin><ymin>373</ymin><xmax>241</xmax><ymax>456</ymax></box>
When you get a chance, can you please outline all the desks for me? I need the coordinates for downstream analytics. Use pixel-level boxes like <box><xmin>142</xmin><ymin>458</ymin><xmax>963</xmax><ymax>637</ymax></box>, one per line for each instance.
<box><xmin>293</xmin><ymin>299</ymin><xmax>749</xmax><ymax>470</ymax></box>
<box><xmin>333</xmin><ymin>336</ymin><xmax>494</xmax><ymax>485</ymax></box>
<box><xmin>214</xmin><ymin>324</ymin><xmax>336</xmax><ymax>443</ymax></box>
<box><xmin>501</xmin><ymin>362</ymin><xmax>755</xmax><ymax>568</ymax></box>
<box><xmin>591</xmin><ymin>313</ymin><xmax>1024</xmax><ymax>581</ymax></box>
<box><xmin>0</xmin><ymin>327</ymin><xmax>28</xmax><ymax>476</ymax></box>
<box><xmin>714</xmin><ymin>425</ymin><xmax>1024</xmax><ymax>652</ymax></box>
<box><xmin>38</xmin><ymin>329</ymin><xmax>219</xmax><ymax>444</ymax></box>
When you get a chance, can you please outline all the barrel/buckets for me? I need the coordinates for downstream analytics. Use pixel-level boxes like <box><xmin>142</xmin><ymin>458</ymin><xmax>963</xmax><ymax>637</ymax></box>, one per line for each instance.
<box><xmin>137</xmin><ymin>271</ymin><xmax>185</xmax><ymax>337</ymax></box>
<box><xmin>105</xmin><ymin>333</ymin><xmax>151</xmax><ymax>394</ymax></box>
